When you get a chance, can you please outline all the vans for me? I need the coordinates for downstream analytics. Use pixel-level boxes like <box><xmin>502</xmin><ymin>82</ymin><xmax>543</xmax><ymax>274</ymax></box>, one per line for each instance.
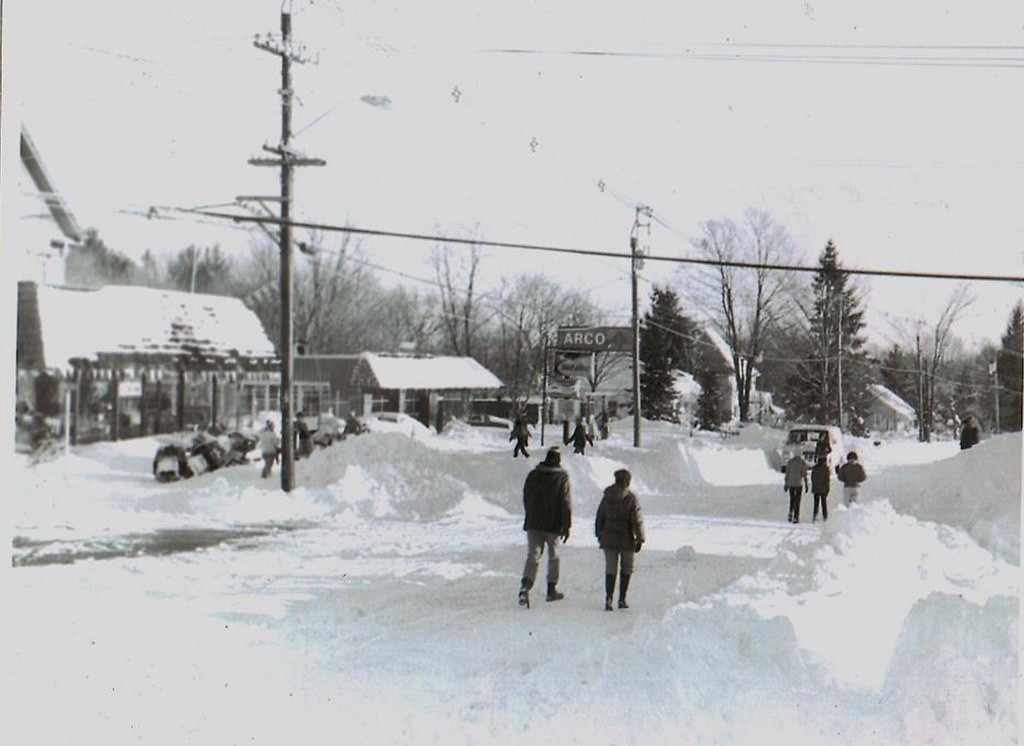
<box><xmin>779</xmin><ymin>423</ymin><xmax>846</xmax><ymax>473</ymax></box>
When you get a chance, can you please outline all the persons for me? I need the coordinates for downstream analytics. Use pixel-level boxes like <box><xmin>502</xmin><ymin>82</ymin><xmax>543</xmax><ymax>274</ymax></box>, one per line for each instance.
<box><xmin>960</xmin><ymin>416</ymin><xmax>981</xmax><ymax>449</ymax></box>
<box><xmin>507</xmin><ymin>413</ymin><xmax>598</xmax><ymax>458</ymax></box>
<box><xmin>519</xmin><ymin>446</ymin><xmax>572</xmax><ymax>608</ymax></box>
<box><xmin>596</xmin><ymin>469</ymin><xmax>645</xmax><ymax>611</ymax></box>
<box><xmin>258</xmin><ymin>406</ymin><xmax>361</xmax><ymax>478</ymax></box>
<box><xmin>783</xmin><ymin>433</ymin><xmax>867</xmax><ymax>524</ymax></box>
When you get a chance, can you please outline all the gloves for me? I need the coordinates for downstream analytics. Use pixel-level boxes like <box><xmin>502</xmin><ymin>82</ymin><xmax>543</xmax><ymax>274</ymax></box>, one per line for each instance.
<box><xmin>635</xmin><ymin>538</ymin><xmax>641</xmax><ymax>552</ymax></box>
<box><xmin>560</xmin><ymin>528</ymin><xmax>568</xmax><ymax>543</ymax></box>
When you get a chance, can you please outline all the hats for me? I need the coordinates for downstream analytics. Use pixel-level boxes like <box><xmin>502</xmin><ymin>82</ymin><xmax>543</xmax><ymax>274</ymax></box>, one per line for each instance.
<box><xmin>615</xmin><ymin>470</ymin><xmax>630</xmax><ymax>487</ymax></box>
<box><xmin>545</xmin><ymin>447</ymin><xmax>561</xmax><ymax>466</ymax></box>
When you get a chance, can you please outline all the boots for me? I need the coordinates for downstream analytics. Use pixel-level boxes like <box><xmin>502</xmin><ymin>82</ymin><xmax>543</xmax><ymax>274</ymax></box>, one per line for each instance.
<box><xmin>547</xmin><ymin>582</ymin><xmax>564</xmax><ymax>601</ymax></box>
<box><xmin>519</xmin><ymin>578</ymin><xmax>534</xmax><ymax>609</ymax></box>
<box><xmin>618</xmin><ymin>574</ymin><xmax>629</xmax><ymax>608</ymax></box>
<box><xmin>605</xmin><ymin>574</ymin><xmax>616</xmax><ymax>610</ymax></box>
